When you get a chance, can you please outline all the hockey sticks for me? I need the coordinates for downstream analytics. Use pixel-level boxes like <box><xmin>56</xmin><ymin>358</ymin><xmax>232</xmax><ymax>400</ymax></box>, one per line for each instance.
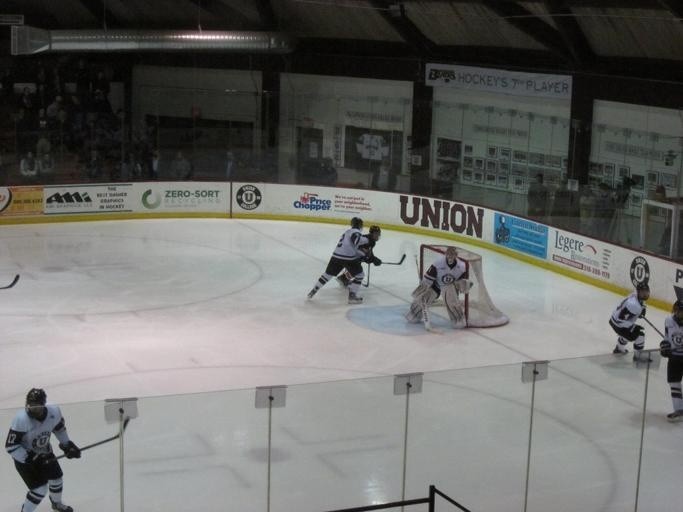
<box><xmin>0</xmin><ymin>275</ymin><xmax>20</xmax><ymax>289</ymax></box>
<box><xmin>370</xmin><ymin>253</ymin><xmax>405</xmax><ymax>265</ymax></box>
<box><xmin>413</xmin><ymin>253</ymin><xmax>442</xmax><ymax>334</ymax></box>
<box><xmin>44</xmin><ymin>416</ymin><xmax>129</xmax><ymax>464</ymax></box>
<box><xmin>361</xmin><ymin>247</ymin><xmax>369</xmax><ymax>288</ymax></box>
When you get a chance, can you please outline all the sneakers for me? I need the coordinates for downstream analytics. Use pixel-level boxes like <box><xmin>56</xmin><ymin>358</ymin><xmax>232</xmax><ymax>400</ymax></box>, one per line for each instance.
<box><xmin>405</xmin><ymin>313</ymin><xmax>465</xmax><ymax>328</ymax></box>
<box><xmin>667</xmin><ymin>410</ymin><xmax>682</xmax><ymax>419</ymax></box>
<box><xmin>307</xmin><ymin>274</ymin><xmax>363</xmax><ymax>302</ymax></box>
<box><xmin>612</xmin><ymin>347</ymin><xmax>655</xmax><ymax>363</ymax></box>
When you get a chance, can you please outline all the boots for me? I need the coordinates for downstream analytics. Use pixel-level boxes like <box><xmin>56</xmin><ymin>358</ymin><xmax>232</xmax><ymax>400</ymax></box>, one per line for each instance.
<box><xmin>49</xmin><ymin>496</ymin><xmax>72</xmax><ymax>512</ymax></box>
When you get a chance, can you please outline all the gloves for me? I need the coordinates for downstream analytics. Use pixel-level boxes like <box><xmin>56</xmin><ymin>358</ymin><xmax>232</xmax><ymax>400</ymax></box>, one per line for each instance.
<box><xmin>659</xmin><ymin>341</ymin><xmax>671</xmax><ymax>356</ymax></box>
<box><xmin>26</xmin><ymin>451</ymin><xmax>49</xmax><ymax>467</ymax></box>
<box><xmin>59</xmin><ymin>442</ymin><xmax>82</xmax><ymax>458</ymax></box>
<box><xmin>366</xmin><ymin>253</ymin><xmax>382</xmax><ymax>266</ymax></box>
<box><xmin>631</xmin><ymin>308</ymin><xmax>647</xmax><ymax>335</ymax></box>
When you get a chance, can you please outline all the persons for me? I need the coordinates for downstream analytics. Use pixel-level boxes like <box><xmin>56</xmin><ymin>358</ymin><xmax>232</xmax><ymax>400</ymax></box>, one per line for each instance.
<box><xmin>526</xmin><ymin>172</ymin><xmax>549</xmax><ymax>216</ymax></box>
<box><xmin>307</xmin><ymin>216</ymin><xmax>365</xmax><ymax>301</ymax></box>
<box><xmin>405</xmin><ymin>246</ymin><xmax>473</xmax><ymax>329</ymax></box>
<box><xmin>167</xmin><ymin>151</ymin><xmax>191</xmax><ymax>182</ymax></box>
<box><xmin>370</xmin><ymin>157</ymin><xmax>398</xmax><ymax>190</ymax></box>
<box><xmin>592</xmin><ymin>182</ymin><xmax>615</xmax><ymax>241</ymax></box>
<box><xmin>412</xmin><ymin>168</ymin><xmax>432</xmax><ymax>196</ymax></box>
<box><xmin>4</xmin><ymin>387</ymin><xmax>80</xmax><ymax>512</ymax></box>
<box><xmin>608</xmin><ymin>282</ymin><xmax>655</xmax><ymax>363</ymax></box>
<box><xmin>302</xmin><ymin>156</ymin><xmax>337</xmax><ymax>187</ymax></box>
<box><xmin>337</xmin><ymin>225</ymin><xmax>381</xmax><ymax>286</ymax></box>
<box><xmin>551</xmin><ymin>177</ymin><xmax>575</xmax><ymax>231</ymax></box>
<box><xmin>659</xmin><ymin>300</ymin><xmax>683</xmax><ymax>418</ymax></box>
<box><xmin>578</xmin><ymin>184</ymin><xmax>595</xmax><ymax>235</ymax></box>
<box><xmin>1</xmin><ymin>56</ymin><xmax>159</xmax><ymax>184</ymax></box>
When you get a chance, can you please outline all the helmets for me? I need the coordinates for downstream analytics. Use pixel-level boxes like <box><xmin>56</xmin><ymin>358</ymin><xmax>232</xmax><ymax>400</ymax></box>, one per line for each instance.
<box><xmin>26</xmin><ymin>390</ymin><xmax>46</xmax><ymax>412</ymax></box>
<box><xmin>350</xmin><ymin>216</ymin><xmax>381</xmax><ymax>236</ymax></box>
<box><xmin>444</xmin><ymin>246</ymin><xmax>457</xmax><ymax>260</ymax></box>
<box><xmin>636</xmin><ymin>281</ymin><xmax>651</xmax><ymax>302</ymax></box>
